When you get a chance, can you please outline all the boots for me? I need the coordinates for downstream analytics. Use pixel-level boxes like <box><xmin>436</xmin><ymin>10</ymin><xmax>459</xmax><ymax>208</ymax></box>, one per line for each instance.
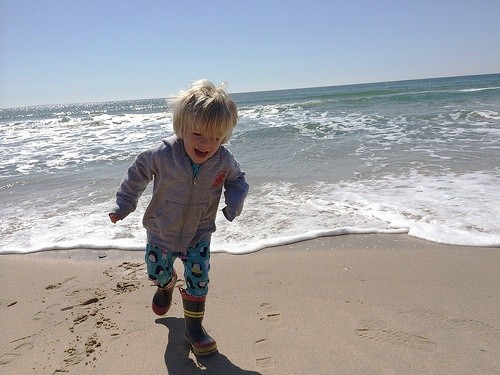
<box><xmin>152</xmin><ymin>269</ymin><xmax>177</xmax><ymax>315</ymax></box>
<box><xmin>179</xmin><ymin>287</ymin><xmax>217</xmax><ymax>356</ymax></box>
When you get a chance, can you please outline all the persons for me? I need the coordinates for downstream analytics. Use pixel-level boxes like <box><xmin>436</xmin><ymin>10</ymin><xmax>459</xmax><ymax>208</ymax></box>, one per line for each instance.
<box><xmin>108</xmin><ymin>78</ymin><xmax>250</xmax><ymax>357</ymax></box>
